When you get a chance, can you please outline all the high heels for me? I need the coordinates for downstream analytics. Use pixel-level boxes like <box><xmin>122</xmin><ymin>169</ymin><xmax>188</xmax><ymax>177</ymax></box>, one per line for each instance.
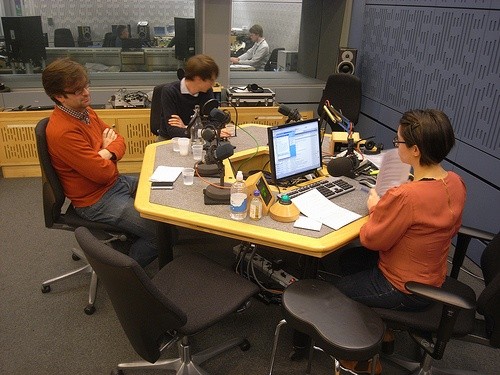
<box><xmin>381</xmin><ymin>330</ymin><xmax>394</xmax><ymax>354</ymax></box>
<box><xmin>340</xmin><ymin>358</ymin><xmax>382</xmax><ymax>375</ymax></box>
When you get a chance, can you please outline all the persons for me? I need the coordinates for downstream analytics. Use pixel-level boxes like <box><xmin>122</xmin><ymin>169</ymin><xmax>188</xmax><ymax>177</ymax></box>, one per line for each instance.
<box><xmin>115</xmin><ymin>25</ymin><xmax>129</xmax><ymax>47</ymax></box>
<box><xmin>230</xmin><ymin>24</ymin><xmax>271</xmax><ymax>72</ymax></box>
<box><xmin>160</xmin><ymin>54</ymin><xmax>220</xmax><ymax>140</ymax></box>
<box><xmin>309</xmin><ymin>108</ymin><xmax>467</xmax><ymax>311</ymax></box>
<box><xmin>42</xmin><ymin>58</ymin><xmax>179</xmax><ymax>278</ymax></box>
<box><xmin>161</xmin><ymin>36</ymin><xmax>176</xmax><ymax>48</ymax></box>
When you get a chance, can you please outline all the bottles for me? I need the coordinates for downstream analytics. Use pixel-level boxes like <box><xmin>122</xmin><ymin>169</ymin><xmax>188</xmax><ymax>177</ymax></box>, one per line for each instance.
<box><xmin>230</xmin><ymin>170</ymin><xmax>248</xmax><ymax>220</ymax></box>
<box><xmin>249</xmin><ymin>190</ymin><xmax>262</xmax><ymax>221</ymax></box>
<box><xmin>191</xmin><ymin>105</ymin><xmax>204</xmax><ymax>147</ymax></box>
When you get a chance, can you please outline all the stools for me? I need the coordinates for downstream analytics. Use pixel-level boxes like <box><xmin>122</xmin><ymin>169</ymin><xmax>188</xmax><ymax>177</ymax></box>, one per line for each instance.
<box><xmin>268</xmin><ymin>279</ymin><xmax>385</xmax><ymax>375</ymax></box>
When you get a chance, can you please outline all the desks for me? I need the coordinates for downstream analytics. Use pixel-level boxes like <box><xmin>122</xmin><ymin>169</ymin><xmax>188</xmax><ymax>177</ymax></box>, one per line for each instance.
<box><xmin>230</xmin><ymin>65</ymin><xmax>257</xmax><ymax>71</ymax></box>
<box><xmin>44</xmin><ymin>46</ymin><xmax>187</xmax><ymax>72</ymax></box>
<box><xmin>133</xmin><ymin>123</ymin><xmax>415</xmax><ymax>362</ymax></box>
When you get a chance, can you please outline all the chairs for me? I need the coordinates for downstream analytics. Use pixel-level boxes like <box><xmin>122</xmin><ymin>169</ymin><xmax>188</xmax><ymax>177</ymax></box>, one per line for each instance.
<box><xmin>264</xmin><ymin>48</ymin><xmax>286</xmax><ymax>71</ymax></box>
<box><xmin>53</xmin><ymin>28</ymin><xmax>117</xmax><ymax>48</ymax></box>
<box><xmin>33</xmin><ymin>73</ymin><xmax>500</xmax><ymax>375</ymax></box>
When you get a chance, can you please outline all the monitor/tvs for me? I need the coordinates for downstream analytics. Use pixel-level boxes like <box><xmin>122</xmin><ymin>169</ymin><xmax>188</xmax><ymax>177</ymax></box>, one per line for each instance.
<box><xmin>1</xmin><ymin>16</ymin><xmax>46</xmax><ymax>59</ymax></box>
<box><xmin>267</xmin><ymin>119</ymin><xmax>323</xmax><ymax>187</ymax></box>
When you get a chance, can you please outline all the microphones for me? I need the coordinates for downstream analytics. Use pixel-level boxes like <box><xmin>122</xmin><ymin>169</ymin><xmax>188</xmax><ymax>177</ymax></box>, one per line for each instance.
<box><xmin>343</xmin><ymin>135</ymin><xmax>376</xmax><ymax>158</ymax></box>
<box><xmin>278</xmin><ymin>104</ymin><xmax>303</xmax><ymax>121</ymax></box>
<box><xmin>202</xmin><ymin>108</ymin><xmax>235</xmax><ymax>165</ymax></box>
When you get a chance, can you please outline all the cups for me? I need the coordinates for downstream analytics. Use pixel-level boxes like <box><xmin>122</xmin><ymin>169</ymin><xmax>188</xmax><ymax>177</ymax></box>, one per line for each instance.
<box><xmin>178</xmin><ymin>138</ymin><xmax>189</xmax><ymax>155</ymax></box>
<box><xmin>192</xmin><ymin>144</ymin><xmax>204</xmax><ymax>161</ymax></box>
<box><xmin>172</xmin><ymin>137</ymin><xmax>181</xmax><ymax>151</ymax></box>
<box><xmin>181</xmin><ymin>168</ymin><xmax>195</xmax><ymax>185</ymax></box>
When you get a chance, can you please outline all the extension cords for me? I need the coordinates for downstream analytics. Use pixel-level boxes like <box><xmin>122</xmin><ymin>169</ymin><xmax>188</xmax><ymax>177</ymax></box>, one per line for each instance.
<box><xmin>232</xmin><ymin>245</ymin><xmax>298</xmax><ymax>288</ymax></box>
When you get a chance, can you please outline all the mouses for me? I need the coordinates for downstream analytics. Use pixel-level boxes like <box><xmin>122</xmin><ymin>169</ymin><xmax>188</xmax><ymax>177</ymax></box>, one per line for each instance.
<box><xmin>359</xmin><ymin>178</ymin><xmax>376</xmax><ymax>188</ymax></box>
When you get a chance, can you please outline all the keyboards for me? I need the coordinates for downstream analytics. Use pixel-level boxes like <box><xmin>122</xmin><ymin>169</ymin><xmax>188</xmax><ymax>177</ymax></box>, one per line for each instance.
<box><xmin>278</xmin><ymin>177</ymin><xmax>355</xmax><ymax>204</ymax></box>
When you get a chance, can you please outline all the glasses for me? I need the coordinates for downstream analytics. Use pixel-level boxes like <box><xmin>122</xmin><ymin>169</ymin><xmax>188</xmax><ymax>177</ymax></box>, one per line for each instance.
<box><xmin>392</xmin><ymin>137</ymin><xmax>412</xmax><ymax>146</ymax></box>
<box><xmin>60</xmin><ymin>80</ymin><xmax>90</xmax><ymax>96</ymax></box>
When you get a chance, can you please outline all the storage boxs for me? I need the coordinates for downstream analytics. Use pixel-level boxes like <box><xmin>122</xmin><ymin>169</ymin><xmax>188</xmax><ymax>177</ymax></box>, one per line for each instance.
<box><xmin>226</xmin><ymin>87</ymin><xmax>275</xmax><ymax>107</ymax></box>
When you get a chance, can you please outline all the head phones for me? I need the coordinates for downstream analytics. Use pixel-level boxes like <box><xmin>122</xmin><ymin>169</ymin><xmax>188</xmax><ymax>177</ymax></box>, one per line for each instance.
<box><xmin>247</xmin><ymin>84</ymin><xmax>264</xmax><ymax>93</ymax></box>
<box><xmin>353</xmin><ymin>140</ymin><xmax>384</xmax><ymax>155</ymax></box>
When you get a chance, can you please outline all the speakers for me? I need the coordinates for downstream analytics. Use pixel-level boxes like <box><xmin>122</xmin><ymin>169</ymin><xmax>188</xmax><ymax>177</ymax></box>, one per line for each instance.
<box><xmin>336</xmin><ymin>48</ymin><xmax>358</xmax><ymax>76</ymax></box>
<box><xmin>137</xmin><ymin>21</ymin><xmax>150</xmax><ymax>39</ymax></box>
<box><xmin>174</xmin><ymin>18</ymin><xmax>196</xmax><ymax>61</ymax></box>
<box><xmin>77</xmin><ymin>26</ymin><xmax>92</xmax><ymax>41</ymax></box>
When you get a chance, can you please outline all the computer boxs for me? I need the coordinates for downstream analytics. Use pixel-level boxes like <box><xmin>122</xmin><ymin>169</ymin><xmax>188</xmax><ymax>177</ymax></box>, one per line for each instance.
<box><xmin>277</xmin><ymin>50</ymin><xmax>299</xmax><ymax>72</ymax></box>
